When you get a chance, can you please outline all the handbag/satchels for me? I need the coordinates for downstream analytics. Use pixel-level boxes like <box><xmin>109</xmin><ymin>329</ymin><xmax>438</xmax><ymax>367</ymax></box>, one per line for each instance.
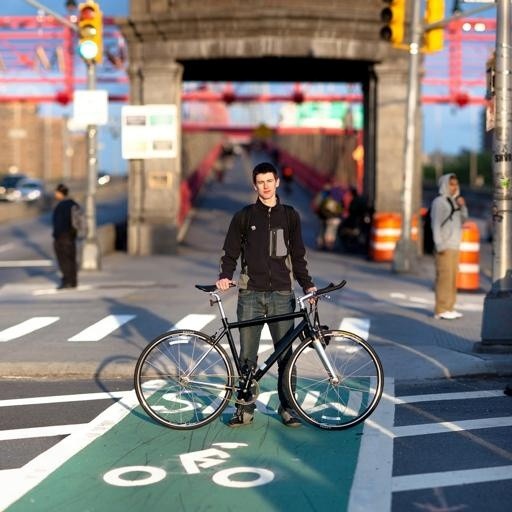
<box><xmin>270</xmin><ymin>228</ymin><xmax>289</xmax><ymax>258</ymax></box>
<box><xmin>71</xmin><ymin>205</ymin><xmax>81</xmax><ymax>231</ymax></box>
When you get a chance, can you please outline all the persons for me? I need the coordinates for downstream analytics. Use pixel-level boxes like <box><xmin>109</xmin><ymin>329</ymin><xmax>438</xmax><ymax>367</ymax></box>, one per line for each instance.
<box><xmin>427</xmin><ymin>170</ymin><xmax>470</xmax><ymax>321</ymax></box>
<box><xmin>214</xmin><ymin>159</ymin><xmax>322</xmax><ymax>427</ymax></box>
<box><xmin>51</xmin><ymin>182</ymin><xmax>82</xmax><ymax>291</ymax></box>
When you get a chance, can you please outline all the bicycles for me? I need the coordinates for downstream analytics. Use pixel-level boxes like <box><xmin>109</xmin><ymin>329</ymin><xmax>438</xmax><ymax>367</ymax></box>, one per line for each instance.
<box><xmin>132</xmin><ymin>278</ymin><xmax>384</xmax><ymax>433</ymax></box>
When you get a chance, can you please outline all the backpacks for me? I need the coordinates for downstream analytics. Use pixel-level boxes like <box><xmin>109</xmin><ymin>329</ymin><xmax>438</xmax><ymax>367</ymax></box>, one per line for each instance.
<box><xmin>423</xmin><ymin>194</ymin><xmax>454</xmax><ymax>253</ymax></box>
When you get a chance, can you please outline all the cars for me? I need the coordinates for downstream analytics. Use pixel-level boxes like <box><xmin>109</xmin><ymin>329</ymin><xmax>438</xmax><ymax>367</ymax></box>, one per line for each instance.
<box><xmin>98</xmin><ymin>171</ymin><xmax>110</xmax><ymax>185</ymax></box>
<box><xmin>12</xmin><ymin>178</ymin><xmax>44</xmax><ymax>204</ymax></box>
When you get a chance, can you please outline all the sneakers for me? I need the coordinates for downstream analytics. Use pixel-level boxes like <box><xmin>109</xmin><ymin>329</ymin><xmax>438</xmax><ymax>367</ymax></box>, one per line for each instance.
<box><xmin>229</xmin><ymin>412</ymin><xmax>254</xmax><ymax>426</ymax></box>
<box><xmin>278</xmin><ymin>404</ymin><xmax>302</xmax><ymax>426</ymax></box>
<box><xmin>435</xmin><ymin>311</ymin><xmax>464</xmax><ymax>319</ymax></box>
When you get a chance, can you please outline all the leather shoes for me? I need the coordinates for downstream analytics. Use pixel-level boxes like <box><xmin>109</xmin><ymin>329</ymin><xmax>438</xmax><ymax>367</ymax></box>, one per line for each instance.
<box><xmin>57</xmin><ymin>281</ymin><xmax>77</xmax><ymax>289</ymax></box>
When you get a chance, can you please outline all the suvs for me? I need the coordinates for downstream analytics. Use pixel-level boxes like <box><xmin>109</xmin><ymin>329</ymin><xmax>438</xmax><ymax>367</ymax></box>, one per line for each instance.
<box><xmin>1</xmin><ymin>173</ymin><xmax>27</xmax><ymax>202</ymax></box>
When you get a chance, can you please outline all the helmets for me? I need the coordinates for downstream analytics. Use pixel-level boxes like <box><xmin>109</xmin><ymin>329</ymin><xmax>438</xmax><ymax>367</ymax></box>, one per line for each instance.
<box><xmin>299</xmin><ymin>325</ymin><xmax>330</xmax><ymax>348</ymax></box>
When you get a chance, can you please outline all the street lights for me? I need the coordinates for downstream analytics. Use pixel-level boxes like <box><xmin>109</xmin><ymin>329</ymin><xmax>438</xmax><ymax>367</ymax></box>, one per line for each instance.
<box><xmin>378</xmin><ymin>1</ymin><xmax>405</xmax><ymax>44</ymax></box>
<box><xmin>77</xmin><ymin>1</ymin><xmax>104</xmax><ymax>65</ymax></box>
